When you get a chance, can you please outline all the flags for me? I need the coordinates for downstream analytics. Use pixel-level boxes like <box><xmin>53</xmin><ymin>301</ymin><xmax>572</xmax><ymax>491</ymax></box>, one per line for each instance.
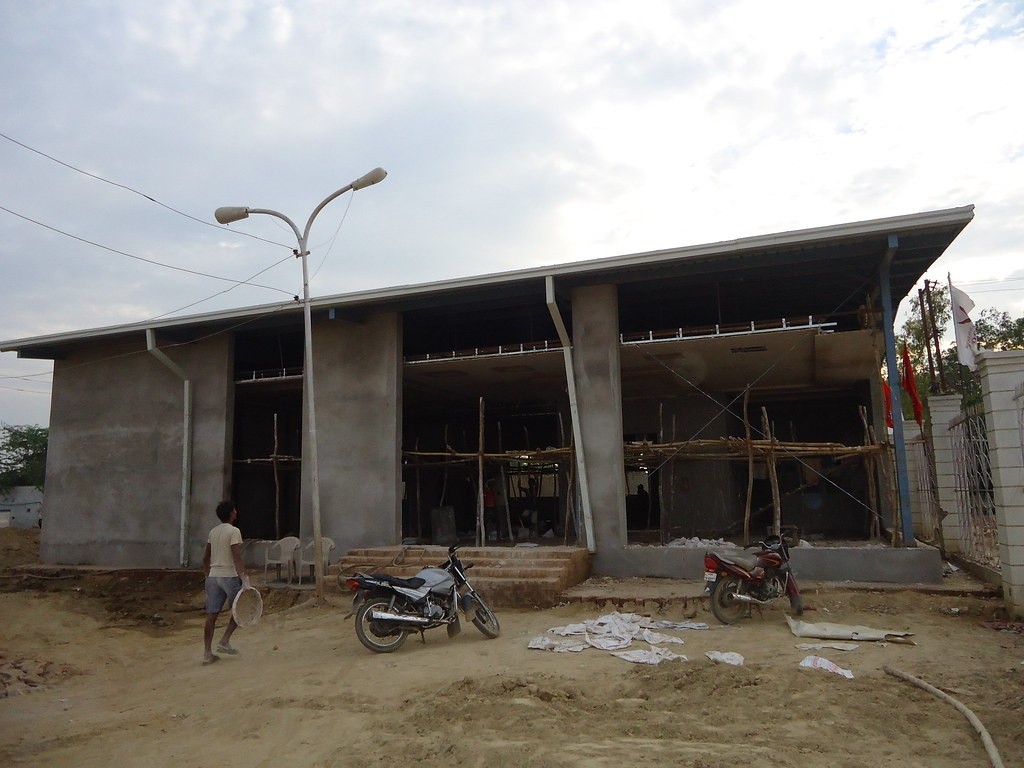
<box><xmin>882</xmin><ymin>383</ymin><xmax>893</xmax><ymax>428</ymax></box>
<box><xmin>902</xmin><ymin>345</ymin><xmax>922</xmax><ymax>425</ymax></box>
<box><xmin>950</xmin><ymin>284</ymin><xmax>978</xmax><ymax>371</ymax></box>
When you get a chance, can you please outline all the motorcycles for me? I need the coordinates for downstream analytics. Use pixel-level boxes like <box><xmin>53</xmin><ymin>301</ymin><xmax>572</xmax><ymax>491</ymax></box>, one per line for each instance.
<box><xmin>704</xmin><ymin>526</ymin><xmax>804</xmax><ymax>624</ymax></box>
<box><xmin>344</xmin><ymin>535</ymin><xmax>500</xmax><ymax>654</ymax></box>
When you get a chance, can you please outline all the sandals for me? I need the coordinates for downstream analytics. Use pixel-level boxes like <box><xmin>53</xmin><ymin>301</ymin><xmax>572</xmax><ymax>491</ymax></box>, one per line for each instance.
<box><xmin>202</xmin><ymin>655</ymin><xmax>219</xmax><ymax>666</ymax></box>
<box><xmin>216</xmin><ymin>644</ymin><xmax>240</xmax><ymax>655</ymax></box>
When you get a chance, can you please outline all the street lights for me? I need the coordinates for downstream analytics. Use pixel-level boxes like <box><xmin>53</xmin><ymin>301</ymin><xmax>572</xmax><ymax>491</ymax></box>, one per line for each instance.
<box><xmin>215</xmin><ymin>166</ymin><xmax>387</xmax><ymax>605</ymax></box>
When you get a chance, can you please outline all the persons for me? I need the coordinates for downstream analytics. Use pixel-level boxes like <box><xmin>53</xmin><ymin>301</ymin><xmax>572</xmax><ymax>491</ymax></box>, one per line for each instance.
<box><xmin>517</xmin><ymin>475</ymin><xmax>539</xmax><ymax>529</ymax></box>
<box><xmin>470</xmin><ymin>479</ymin><xmax>497</xmax><ymax>541</ymax></box>
<box><xmin>637</xmin><ymin>485</ymin><xmax>649</xmax><ymax>530</ymax></box>
<box><xmin>202</xmin><ymin>502</ymin><xmax>251</xmax><ymax>666</ymax></box>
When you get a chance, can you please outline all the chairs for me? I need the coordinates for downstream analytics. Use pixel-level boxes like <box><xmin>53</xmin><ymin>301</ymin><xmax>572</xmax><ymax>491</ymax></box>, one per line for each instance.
<box><xmin>298</xmin><ymin>537</ymin><xmax>336</xmax><ymax>585</ymax></box>
<box><xmin>263</xmin><ymin>537</ymin><xmax>302</xmax><ymax>583</ymax></box>
